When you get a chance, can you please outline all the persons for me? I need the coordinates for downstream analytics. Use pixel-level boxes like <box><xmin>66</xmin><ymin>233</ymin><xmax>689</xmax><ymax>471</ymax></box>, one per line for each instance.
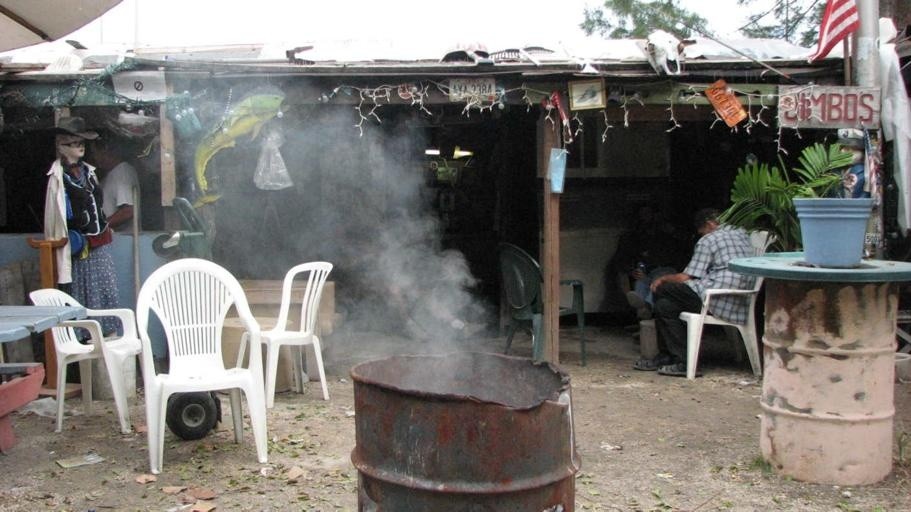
<box><xmin>650</xmin><ymin>207</ymin><xmax>760</xmax><ymax>375</ymax></box>
<box><xmin>42</xmin><ymin>115</ymin><xmax>122</xmax><ymax>341</ymax></box>
<box><xmin>88</xmin><ymin>134</ymin><xmax>141</xmax><ymax>232</ymax></box>
<box><xmin>825</xmin><ymin>137</ymin><xmax>871</xmax><ymax>198</ymax></box>
<box><xmin>617</xmin><ymin>199</ymin><xmax>681</xmax><ymax>319</ymax></box>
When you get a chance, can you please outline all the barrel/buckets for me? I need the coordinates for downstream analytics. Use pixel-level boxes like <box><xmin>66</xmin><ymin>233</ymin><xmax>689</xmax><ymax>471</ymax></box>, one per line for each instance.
<box><xmin>350</xmin><ymin>354</ymin><xmax>582</xmax><ymax>512</ymax></box>
<box><xmin>759</xmin><ymin>279</ymin><xmax>899</xmax><ymax>483</ymax></box>
<box><xmin>794</xmin><ymin>198</ymin><xmax>877</xmax><ymax>266</ymax></box>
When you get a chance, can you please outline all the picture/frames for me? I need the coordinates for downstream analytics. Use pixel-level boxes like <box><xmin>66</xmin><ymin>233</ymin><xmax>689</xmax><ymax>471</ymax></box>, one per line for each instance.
<box><xmin>565</xmin><ymin>78</ymin><xmax>607</xmax><ymax>111</ymax></box>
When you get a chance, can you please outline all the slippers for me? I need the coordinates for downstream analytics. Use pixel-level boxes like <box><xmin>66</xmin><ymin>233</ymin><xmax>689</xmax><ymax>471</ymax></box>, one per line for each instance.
<box><xmin>633</xmin><ymin>353</ymin><xmax>704</xmax><ymax>377</ymax></box>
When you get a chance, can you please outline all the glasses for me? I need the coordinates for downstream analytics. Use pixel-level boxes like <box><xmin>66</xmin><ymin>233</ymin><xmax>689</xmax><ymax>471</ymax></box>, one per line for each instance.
<box><xmin>61</xmin><ymin>140</ymin><xmax>88</xmax><ymax>148</ymax></box>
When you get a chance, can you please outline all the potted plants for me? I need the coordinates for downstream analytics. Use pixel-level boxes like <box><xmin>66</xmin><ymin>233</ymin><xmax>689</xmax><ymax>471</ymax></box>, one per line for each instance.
<box><xmin>722</xmin><ymin>134</ymin><xmax>878</xmax><ymax>268</ymax></box>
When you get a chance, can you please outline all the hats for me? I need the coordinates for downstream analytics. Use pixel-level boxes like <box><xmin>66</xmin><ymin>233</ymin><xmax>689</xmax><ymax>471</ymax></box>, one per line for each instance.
<box><xmin>45</xmin><ymin>117</ymin><xmax>99</xmax><ymax>141</ymax></box>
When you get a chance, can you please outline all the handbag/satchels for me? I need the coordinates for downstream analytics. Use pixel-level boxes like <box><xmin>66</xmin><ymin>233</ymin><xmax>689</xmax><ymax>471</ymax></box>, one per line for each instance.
<box><xmin>86</xmin><ymin>228</ymin><xmax>113</xmax><ymax>249</ymax></box>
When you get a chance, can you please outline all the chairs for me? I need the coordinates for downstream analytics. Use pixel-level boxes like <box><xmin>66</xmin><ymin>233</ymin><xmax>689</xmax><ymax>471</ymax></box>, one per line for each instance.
<box><xmin>28</xmin><ymin>286</ymin><xmax>144</xmax><ymax>438</ymax></box>
<box><xmin>233</xmin><ymin>258</ymin><xmax>333</xmax><ymax>407</ymax></box>
<box><xmin>670</xmin><ymin>223</ymin><xmax>778</xmax><ymax>382</ymax></box>
<box><xmin>492</xmin><ymin>239</ymin><xmax>588</xmax><ymax>369</ymax></box>
<box><xmin>134</xmin><ymin>257</ymin><xmax>268</xmax><ymax>475</ymax></box>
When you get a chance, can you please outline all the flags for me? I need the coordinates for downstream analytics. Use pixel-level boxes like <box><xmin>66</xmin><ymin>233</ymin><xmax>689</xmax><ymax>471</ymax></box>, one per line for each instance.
<box><xmin>805</xmin><ymin>0</ymin><xmax>860</xmax><ymax>62</ymax></box>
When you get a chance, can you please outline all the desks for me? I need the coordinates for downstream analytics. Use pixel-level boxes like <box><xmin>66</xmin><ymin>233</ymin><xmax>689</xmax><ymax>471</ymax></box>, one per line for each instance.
<box><xmin>0</xmin><ymin>302</ymin><xmax>87</xmax><ymax>458</ymax></box>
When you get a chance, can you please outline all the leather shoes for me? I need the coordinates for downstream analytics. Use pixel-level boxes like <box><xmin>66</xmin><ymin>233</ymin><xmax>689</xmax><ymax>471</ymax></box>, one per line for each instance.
<box><xmin>626</xmin><ymin>290</ymin><xmax>653</xmax><ymax>320</ymax></box>
